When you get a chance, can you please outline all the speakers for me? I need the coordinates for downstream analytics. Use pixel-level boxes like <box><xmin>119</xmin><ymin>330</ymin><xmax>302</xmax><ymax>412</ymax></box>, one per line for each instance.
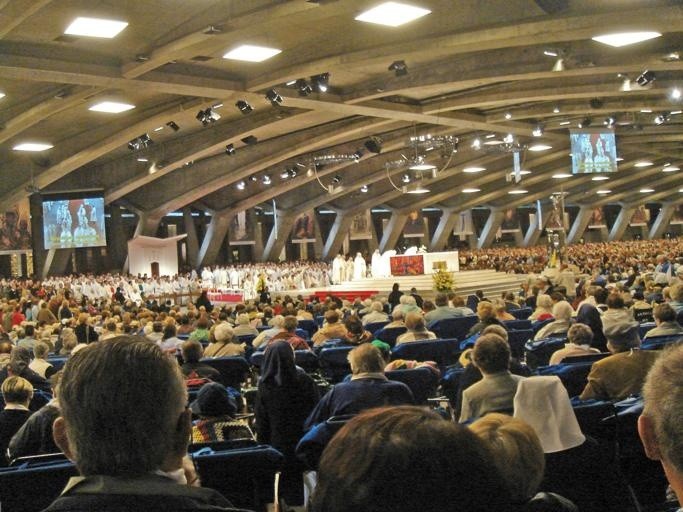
<box><xmin>364</xmin><ymin>140</ymin><xmax>382</xmax><ymax>153</ymax></box>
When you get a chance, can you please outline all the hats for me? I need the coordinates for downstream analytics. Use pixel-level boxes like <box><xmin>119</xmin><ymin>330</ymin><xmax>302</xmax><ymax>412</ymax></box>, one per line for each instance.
<box><xmin>188</xmin><ymin>382</ymin><xmax>238</xmax><ymax>416</ymax></box>
<box><xmin>603</xmin><ymin>321</ymin><xmax>641</xmax><ymax>344</ymax></box>
<box><xmin>632</xmin><ymin>293</ymin><xmax>644</xmax><ymax>299</ymax></box>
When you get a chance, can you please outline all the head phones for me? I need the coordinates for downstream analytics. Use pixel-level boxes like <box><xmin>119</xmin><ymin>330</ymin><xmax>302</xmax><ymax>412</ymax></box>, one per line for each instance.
<box><xmin>175</xmin><ymin>401</ymin><xmax>193</xmax><ymax>456</ymax></box>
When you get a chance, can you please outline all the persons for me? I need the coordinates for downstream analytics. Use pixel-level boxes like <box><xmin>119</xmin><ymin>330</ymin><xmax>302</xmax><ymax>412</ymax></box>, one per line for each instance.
<box><xmin>0</xmin><ymin>212</ymin><xmax>32</xmax><ymax>251</ymax></box>
<box><xmin>37</xmin><ymin>335</ymin><xmax>261</xmax><ymax>512</ymax></box>
<box><xmin>637</xmin><ymin>339</ymin><xmax>682</xmax><ymax>512</ymax></box>
<box><xmin>1</xmin><ymin>233</ymin><xmax>682</xmax><ymax>466</ymax></box>
<box><xmin>306</xmin><ymin>405</ymin><xmax>496</xmax><ymax>512</ymax></box>
<box><xmin>49</xmin><ymin>202</ymin><xmax>101</xmax><ymax>248</ymax></box>
<box><xmin>227</xmin><ymin>201</ymin><xmax>683</xmax><ymax>244</ymax></box>
<box><xmin>469</xmin><ymin>411</ymin><xmax>579</xmax><ymax>512</ymax></box>
<box><xmin>578</xmin><ymin>136</ymin><xmax>618</xmax><ymax>175</ymax></box>
